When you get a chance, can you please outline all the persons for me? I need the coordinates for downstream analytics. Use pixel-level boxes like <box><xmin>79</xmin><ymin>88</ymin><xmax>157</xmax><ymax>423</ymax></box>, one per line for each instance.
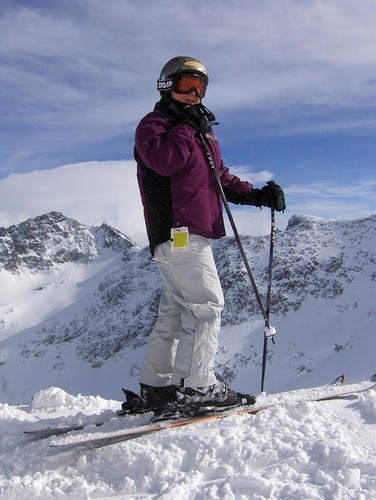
<box><xmin>134</xmin><ymin>55</ymin><xmax>286</xmax><ymax>420</ymax></box>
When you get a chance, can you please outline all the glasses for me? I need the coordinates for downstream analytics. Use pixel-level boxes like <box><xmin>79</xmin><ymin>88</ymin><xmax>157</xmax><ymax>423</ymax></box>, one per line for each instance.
<box><xmin>156</xmin><ymin>73</ymin><xmax>207</xmax><ymax>98</ymax></box>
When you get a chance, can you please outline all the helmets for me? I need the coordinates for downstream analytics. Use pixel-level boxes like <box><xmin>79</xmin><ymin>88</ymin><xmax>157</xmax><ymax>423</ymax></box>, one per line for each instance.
<box><xmin>158</xmin><ymin>55</ymin><xmax>209</xmax><ymax>97</ymax></box>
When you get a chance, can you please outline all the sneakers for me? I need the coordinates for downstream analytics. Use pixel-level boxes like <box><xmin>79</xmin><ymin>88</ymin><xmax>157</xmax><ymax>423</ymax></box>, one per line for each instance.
<box><xmin>177</xmin><ymin>379</ymin><xmax>257</xmax><ymax>412</ymax></box>
<box><xmin>140</xmin><ymin>378</ymin><xmax>186</xmax><ymax>399</ymax></box>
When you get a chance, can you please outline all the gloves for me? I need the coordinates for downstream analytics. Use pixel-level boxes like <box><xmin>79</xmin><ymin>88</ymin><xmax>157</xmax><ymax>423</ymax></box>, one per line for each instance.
<box><xmin>175</xmin><ymin>104</ymin><xmax>216</xmax><ymax>141</ymax></box>
<box><xmin>256</xmin><ymin>185</ymin><xmax>286</xmax><ymax>213</ymax></box>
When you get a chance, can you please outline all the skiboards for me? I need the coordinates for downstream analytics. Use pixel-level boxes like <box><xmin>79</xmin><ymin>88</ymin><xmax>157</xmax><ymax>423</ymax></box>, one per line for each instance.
<box><xmin>24</xmin><ymin>375</ymin><xmax>346</xmax><ymax>436</ymax></box>
<box><xmin>49</xmin><ymin>374</ymin><xmax>376</xmax><ymax>449</ymax></box>
